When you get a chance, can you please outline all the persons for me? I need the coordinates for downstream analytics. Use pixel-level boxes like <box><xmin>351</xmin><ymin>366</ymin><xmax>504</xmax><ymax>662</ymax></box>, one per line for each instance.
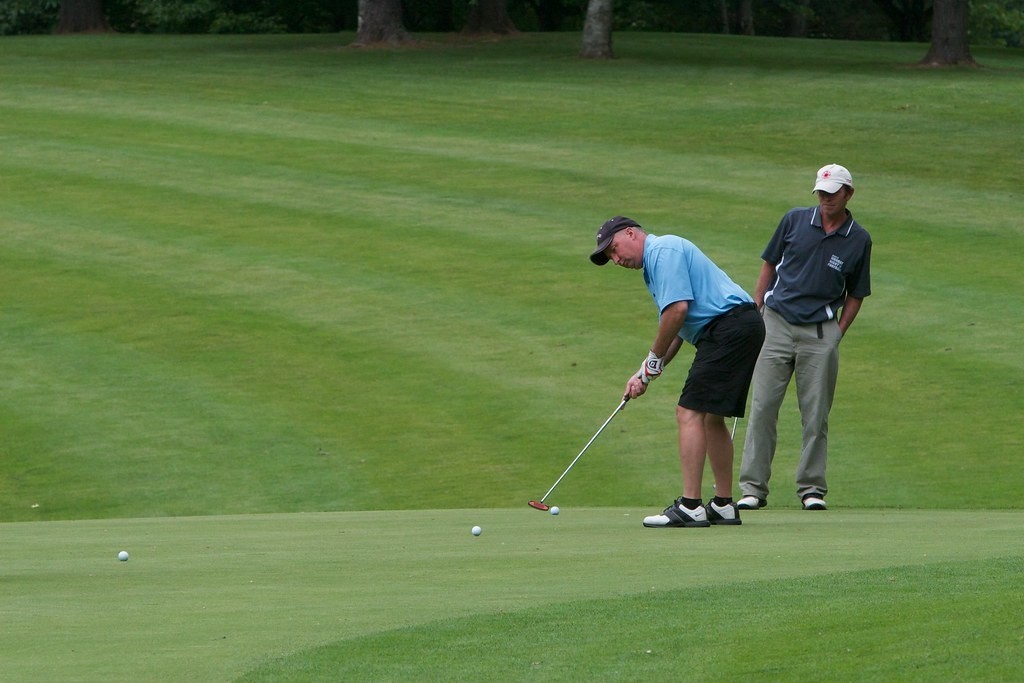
<box><xmin>752</xmin><ymin>162</ymin><xmax>872</xmax><ymax>510</ymax></box>
<box><xmin>590</xmin><ymin>215</ymin><xmax>767</xmax><ymax>528</ymax></box>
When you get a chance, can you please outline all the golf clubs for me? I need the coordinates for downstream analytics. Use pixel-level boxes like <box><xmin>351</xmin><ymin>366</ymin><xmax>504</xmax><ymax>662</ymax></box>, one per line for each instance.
<box><xmin>527</xmin><ymin>394</ymin><xmax>630</xmax><ymax>511</ymax></box>
<box><xmin>714</xmin><ymin>416</ymin><xmax>738</xmax><ymax>489</ymax></box>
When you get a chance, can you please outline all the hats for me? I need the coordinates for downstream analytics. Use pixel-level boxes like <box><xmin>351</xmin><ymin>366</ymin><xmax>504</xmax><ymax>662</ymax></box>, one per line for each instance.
<box><xmin>589</xmin><ymin>215</ymin><xmax>641</xmax><ymax>267</ymax></box>
<box><xmin>812</xmin><ymin>163</ymin><xmax>854</xmax><ymax>196</ymax></box>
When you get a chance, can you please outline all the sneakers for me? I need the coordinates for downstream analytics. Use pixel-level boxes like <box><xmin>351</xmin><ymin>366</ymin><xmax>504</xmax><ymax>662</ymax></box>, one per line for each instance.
<box><xmin>642</xmin><ymin>503</ymin><xmax>742</xmax><ymax>528</ymax></box>
<box><xmin>801</xmin><ymin>494</ymin><xmax>828</xmax><ymax>510</ymax></box>
<box><xmin>735</xmin><ymin>496</ymin><xmax>768</xmax><ymax>510</ymax></box>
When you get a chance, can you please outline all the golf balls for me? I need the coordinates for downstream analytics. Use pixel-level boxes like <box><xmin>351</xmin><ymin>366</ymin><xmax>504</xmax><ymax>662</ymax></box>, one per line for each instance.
<box><xmin>550</xmin><ymin>506</ymin><xmax>560</xmax><ymax>515</ymax></box>
<box><xmin>118</xmin><ymin>551</ymin><xmax>128</xmax><ymax>561</ymax></box>
<box><xmin>472</xmin><ymin>526</ymin><xmax>482</xmax><ymax>536</ymax></box>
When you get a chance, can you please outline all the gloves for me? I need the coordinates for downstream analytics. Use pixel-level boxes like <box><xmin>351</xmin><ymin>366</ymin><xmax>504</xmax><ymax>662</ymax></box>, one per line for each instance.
<box><xmin>635</xmin><ymin>350</ymin><xmax>666</xmax><ymax>386</ymax></box>
